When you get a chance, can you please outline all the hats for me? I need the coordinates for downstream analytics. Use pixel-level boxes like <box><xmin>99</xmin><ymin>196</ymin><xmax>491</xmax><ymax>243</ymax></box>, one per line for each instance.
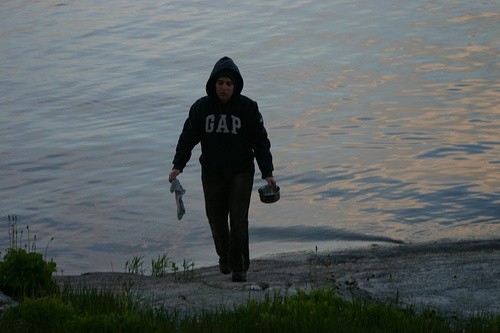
<box><xmin>212</xmin><ymin>69</ymin><xmax>238</xmax><ymax>94</ymax></box>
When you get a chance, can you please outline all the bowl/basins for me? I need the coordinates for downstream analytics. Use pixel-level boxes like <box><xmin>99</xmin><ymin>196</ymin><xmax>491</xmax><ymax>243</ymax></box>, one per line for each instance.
<box><xmin>258</xmin><ymin>185</ymin><xmax>280</xmax><ymax>204</ymax></box>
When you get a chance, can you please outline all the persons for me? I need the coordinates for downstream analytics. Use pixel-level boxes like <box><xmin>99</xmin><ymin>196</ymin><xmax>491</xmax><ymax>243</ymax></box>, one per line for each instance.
<box><xmin>168</xmin><ymin>56</ymin><xmax>276</xmax><ymax>282</ymax></box>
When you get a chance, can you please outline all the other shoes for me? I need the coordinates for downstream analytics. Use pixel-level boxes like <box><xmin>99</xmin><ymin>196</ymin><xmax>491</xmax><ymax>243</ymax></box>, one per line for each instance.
<box><xmin>231</xmin><ymin>272</ymin><xmax>247</xmax><ymax>282</ymax></box>
<box><xmin>220</xmin><ymin>265</ymin><xmax>232</xmax><ymax>274</ymax></box>
